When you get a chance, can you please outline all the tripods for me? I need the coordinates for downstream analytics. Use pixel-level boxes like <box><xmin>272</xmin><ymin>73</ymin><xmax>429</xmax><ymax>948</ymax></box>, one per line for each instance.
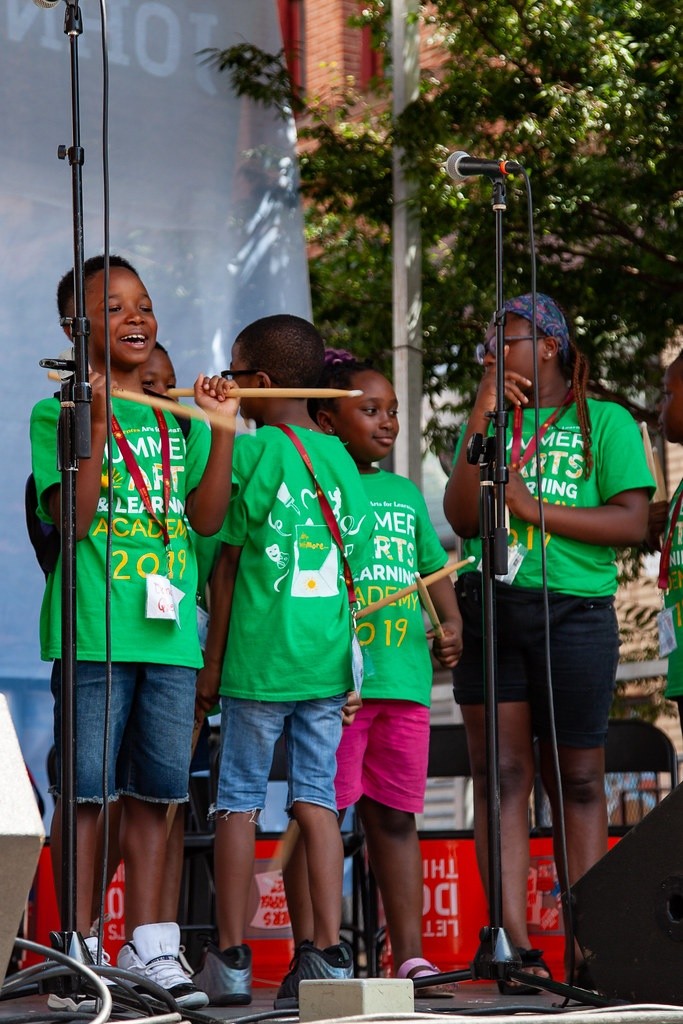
<box><xmin>412</xmin><ymin>174</ymin><xmax>604</xmax><ymax>1006</ymax></box>
<box><xmin>0</xmin><ymin>0</ymin><xmax>228</xmax><ymax>1024</ymax></box>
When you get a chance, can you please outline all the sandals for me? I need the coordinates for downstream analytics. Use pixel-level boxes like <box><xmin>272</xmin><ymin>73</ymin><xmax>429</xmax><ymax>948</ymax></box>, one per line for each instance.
<box><xmin>397</xmin><ymin>958</ymin><xmax>456</xmax><ymax>998</ymax></box>
<box><xmin>497</xmin><ymin>947</ymin><xmax>551</xmax><ymax>995</ymax></box>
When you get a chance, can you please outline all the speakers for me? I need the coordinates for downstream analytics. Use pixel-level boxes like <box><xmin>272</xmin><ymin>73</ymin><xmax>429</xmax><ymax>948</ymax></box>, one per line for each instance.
<box><xmin>561</xmin><ymin>779</ymin><xmax>683</xmax><ymax>1006</ymax></box>
<box><xmin>0</xmin><ymin>692</ymin><xmax>47</xmax><ymax>978</ymax></box>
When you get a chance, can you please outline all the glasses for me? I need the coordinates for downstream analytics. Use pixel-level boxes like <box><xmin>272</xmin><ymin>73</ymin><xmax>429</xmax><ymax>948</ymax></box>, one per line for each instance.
<box><xmin>476</xmin><ymin>334</ymin><xmax>545</xmax><ymax>365</ymax></box>
<box><xmin>221</xmin><ymin>370</ymin><xmax>281</xmax><ymax>385</ymax></box>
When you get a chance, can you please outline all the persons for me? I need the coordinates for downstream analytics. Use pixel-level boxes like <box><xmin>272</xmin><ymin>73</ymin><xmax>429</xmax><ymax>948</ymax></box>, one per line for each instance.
<box><xmin>195</xmin><ymin>315</ymin><xmax>377</xmax><ymax>1008</ymax></box>
<box><xmin>444</xmin><ymin>290</ymin><xmax>683</xmax><ymax>996</ymax></box>
<box><xmin>282</xmin><ymin>361</ymin><xmax>463</xmax><ymax>979</ymax></box>
<box><xmin>90</xmin><ymin>341</ymin><xmax>186</xmax><ymax>924</ymax></box>
<box><xmin>28</xmin><ymin>256</ymin><xmax>240</xmax><ymax>1013</ymax></box>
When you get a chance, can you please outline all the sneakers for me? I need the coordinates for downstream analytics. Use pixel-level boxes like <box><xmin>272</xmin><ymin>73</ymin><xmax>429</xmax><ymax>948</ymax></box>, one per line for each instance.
<box><xmin>46</xmin><ymin>936</ymin><xmax>128</xmax><ymax>1013</ymax></box>
<box><xmin>274</xmin><ymin>936</ymin><xmax>354</xmax><ymax>1008</ymax></box>
<box><xmin>188</xmin><ymin>933</ymin><xmax>252</xmax><ymax>1006</ymax></box>
<box><xmin>117</xmin><ymin>922</ymin><xmax>209</xmax><ymax>1010</ymax></box>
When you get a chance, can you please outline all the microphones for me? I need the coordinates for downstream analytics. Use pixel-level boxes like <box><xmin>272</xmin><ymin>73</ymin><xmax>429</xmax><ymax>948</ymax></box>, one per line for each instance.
<box><xmin>33</xmin><ymin>0</ymin><xmax>61</xmax><ymax>9</ymax></box>
<box><xmin>445</xmin><ymin>150</ymin><xmax>522</xmax><ymax>183</ymax></box>
